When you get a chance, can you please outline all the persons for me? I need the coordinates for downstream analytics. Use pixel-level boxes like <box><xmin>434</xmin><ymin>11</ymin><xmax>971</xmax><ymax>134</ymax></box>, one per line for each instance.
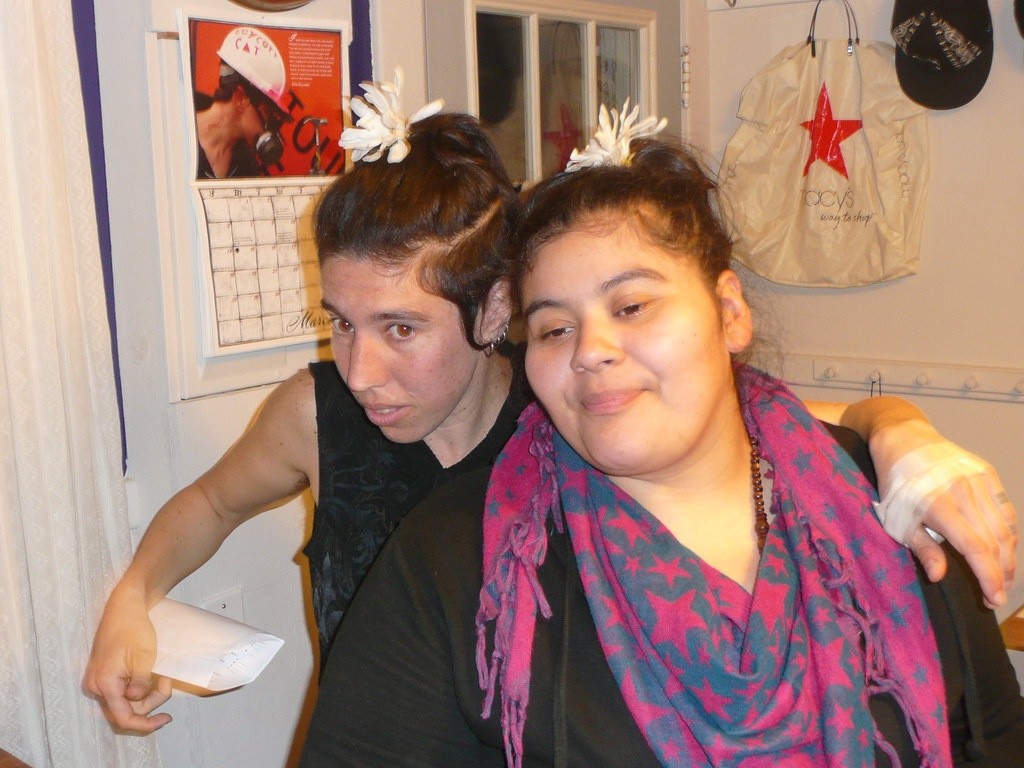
<box><xmin>77</xmin><ymin>65</ymin><xmax>1018</xmax><ymax>768</ymax></box>
<box><xmin>195</xmin><ymin>26</ymin><xmax>290</xmax><ymax>178</ymax></box>
<box><xmin>296</xmin><ymin>94</ymin><xmax>1024</xmax><ymax>768</ymax></box>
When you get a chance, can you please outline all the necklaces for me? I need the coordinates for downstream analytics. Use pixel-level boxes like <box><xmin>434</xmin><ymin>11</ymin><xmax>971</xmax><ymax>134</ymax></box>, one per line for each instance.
<box><xmin>749</xmin><ymin>434</ymin><xmax>769</xmax><ymax>554</ymax></box>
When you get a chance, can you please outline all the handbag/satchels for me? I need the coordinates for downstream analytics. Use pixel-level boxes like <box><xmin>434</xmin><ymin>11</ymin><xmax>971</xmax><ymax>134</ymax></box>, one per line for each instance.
<box><xmin>717</xmin><ymin>0</ymin><xmax>929</xmax><ymax>289</ymax></box>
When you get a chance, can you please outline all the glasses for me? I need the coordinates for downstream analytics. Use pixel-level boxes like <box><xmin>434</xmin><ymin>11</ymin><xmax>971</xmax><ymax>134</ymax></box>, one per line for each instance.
<box><xmin>252</xmin><ymin>102</ymin><xmax>283</xmax><ymax>134</ymax></box>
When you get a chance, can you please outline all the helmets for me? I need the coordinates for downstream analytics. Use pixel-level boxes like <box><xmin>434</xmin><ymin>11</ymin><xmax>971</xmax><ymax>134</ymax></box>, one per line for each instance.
<box><xmin>216</xmin><ymin>27</ymin><xmax>289</xmax><ymax>116</ymax></box>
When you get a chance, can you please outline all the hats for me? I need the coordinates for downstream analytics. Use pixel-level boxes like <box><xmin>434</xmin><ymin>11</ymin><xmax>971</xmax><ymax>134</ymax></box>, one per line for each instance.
<box><xmin>890</xmin><ymin>0</ymin><xmax>994</xmax><ymax>111</ymax></box>
